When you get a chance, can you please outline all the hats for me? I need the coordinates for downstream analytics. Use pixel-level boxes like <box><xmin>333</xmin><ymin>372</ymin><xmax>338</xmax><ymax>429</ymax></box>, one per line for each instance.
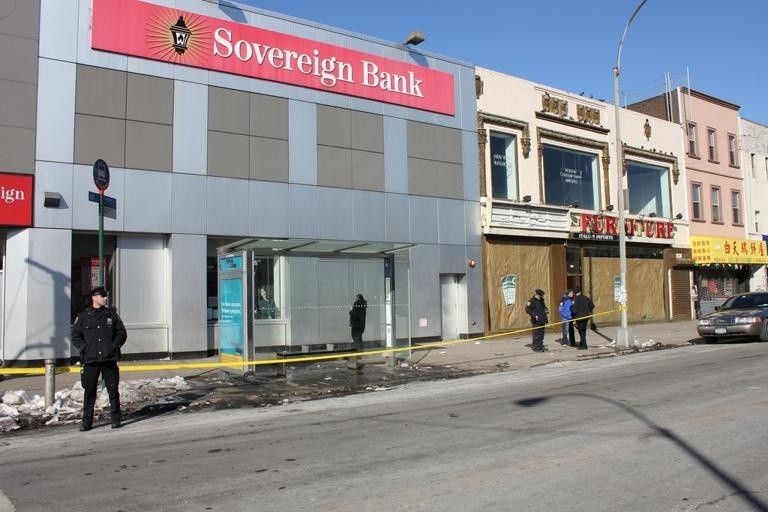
<box><xmin>574</xmin><ymin>287</ymin><xmax>580</xmax><ymax>293</ymax></box>
<box><xmin>535</xmin><ymin>290</ymin><xmax>544</xmax><ymax>296</ymax></box>
<box><xmin>89</xmin><ymin>287</ymin><xmax>106</xmax><ymax>296</ymax></box>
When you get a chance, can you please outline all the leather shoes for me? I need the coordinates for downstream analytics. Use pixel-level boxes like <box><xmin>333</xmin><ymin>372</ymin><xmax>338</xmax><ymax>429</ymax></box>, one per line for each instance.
<box><xmin>534</xmin><ymin>348</ymin><xmax>543</xmax><ymax>352</ymax></box>
<box><xmin>111</xmin><ymin>422</ymin><xmax>120</xmax><ymax>428</ymax></box>
<box><xmin>80</xmin><ymin>424</ymin><xmax>92</xmax><ymax>431</ymax></box>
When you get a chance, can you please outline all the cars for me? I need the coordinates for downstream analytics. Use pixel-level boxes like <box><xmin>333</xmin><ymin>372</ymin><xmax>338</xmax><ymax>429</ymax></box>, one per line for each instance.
<box><xmin>697</xmin><ymin>291</ymin><xmax>768</xmax><ymax>344</ymax></box>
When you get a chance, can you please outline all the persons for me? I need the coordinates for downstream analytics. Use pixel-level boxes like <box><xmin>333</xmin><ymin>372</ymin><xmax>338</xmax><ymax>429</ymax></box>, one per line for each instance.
<box><xmin>690</xmin><ymin>286</ymin><xmax>697</xmax><ymax>296</ymax></box>
<box><xmin>348</xmin><ymin>293</ymin><xmax>368</xmax><ymax>354</ymax></box>
<box><xmin>524</xmin><ymin>289</ymin><xmax>550</xmax><ymax>353</ymax></box>
<box><xmin>257</xmin><ymin>286</ymin><xmax>278</xmax><ymax>320</ymax></box>
<box><xmin>556</xmin><ymin>288</ymin><xmax>579</xmax><ymax>348</ymax></box>
<box><xmin>569</xmin><ymin>288</ymin><xmax>595</xmax><ymax>351</ymax></box>
<box><xmin>68</xmin><ymin>284</ymin><xmax>129</xmax><ymax>431</ymax></box>
